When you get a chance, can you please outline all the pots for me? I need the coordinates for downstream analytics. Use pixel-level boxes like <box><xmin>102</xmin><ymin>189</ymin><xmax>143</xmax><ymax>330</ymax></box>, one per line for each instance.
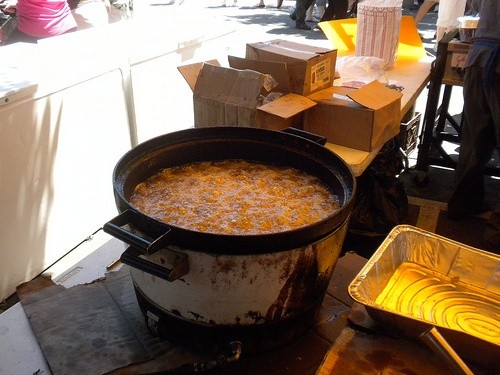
<box><xmin>103</xmin><ymin>126</ymin><xmax>357</xmax><ymax>359</ymax></box>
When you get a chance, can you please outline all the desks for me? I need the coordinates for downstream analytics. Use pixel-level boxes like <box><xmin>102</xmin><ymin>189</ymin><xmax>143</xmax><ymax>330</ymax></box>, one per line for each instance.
<box><xmin>419</xmin><ymin>32</ymin><xmax>494</xmax><ymax>169</ymax></box>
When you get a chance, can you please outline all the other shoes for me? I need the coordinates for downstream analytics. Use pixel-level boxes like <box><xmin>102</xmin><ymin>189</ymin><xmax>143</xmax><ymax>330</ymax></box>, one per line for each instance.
<box><xmin>296</xmin><ymin>24</ymin><xmax>311</xmax><ymax>30</ymax></box>
<box><xmin>289</xmin><ymin>13</ymin><xmax>297</xmax><ymax>20</ymax></box>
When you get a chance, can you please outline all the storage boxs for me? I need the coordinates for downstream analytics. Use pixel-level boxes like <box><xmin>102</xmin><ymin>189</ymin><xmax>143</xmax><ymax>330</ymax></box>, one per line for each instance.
<box><xmin>177</xmin><ymin>55</ymin><xmax>318</xmax><ymax>131</ymax></box>
<box><xmin>246</xmin><ymin>39</ymin><xmax>338</xmax><ymax>97</ymax></box>
<box><xmin>307</xmin><ymin>79</ymin><xmax>403</xmax><ymax>152</ymax></box>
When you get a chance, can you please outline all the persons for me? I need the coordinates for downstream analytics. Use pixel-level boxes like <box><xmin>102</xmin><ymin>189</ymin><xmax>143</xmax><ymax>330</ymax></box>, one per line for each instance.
<box><xmin>1</xmin><ymin>0</ymin><xmax>78</xmax><ymax>44</ymax></box>
<box><xmin>455</xmin><ymin>0</ymin><xmax>500</xmax><ymax>226</ymax></box>
<box><xmin>290</xmin><ymin>0</ymin><xmax>356</xmax><ymax>33</ymax></box>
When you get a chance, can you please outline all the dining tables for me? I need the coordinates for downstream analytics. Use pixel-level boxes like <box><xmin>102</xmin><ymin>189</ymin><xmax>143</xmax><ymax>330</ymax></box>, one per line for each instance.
<box><xmin>0</xmin><ymin>237</ymin><xmax>370</xmax><ymax>375</ymax></box>
<box><xmin>311</xmin><ymin>61</ymin><xmax>436</xmax><ymax>177</ymax></box>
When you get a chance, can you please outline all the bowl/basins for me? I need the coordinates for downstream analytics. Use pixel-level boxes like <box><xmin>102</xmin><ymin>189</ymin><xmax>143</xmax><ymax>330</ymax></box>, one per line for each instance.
<box><xmin>347</xmin><ymin>224</ymin><xmax>500</xmax><ymax>365</ymax></box>
<box><xmin>459</xmin><ymin>27</ymin><xmax>477</xmax><ymax>43</ymax></box>
